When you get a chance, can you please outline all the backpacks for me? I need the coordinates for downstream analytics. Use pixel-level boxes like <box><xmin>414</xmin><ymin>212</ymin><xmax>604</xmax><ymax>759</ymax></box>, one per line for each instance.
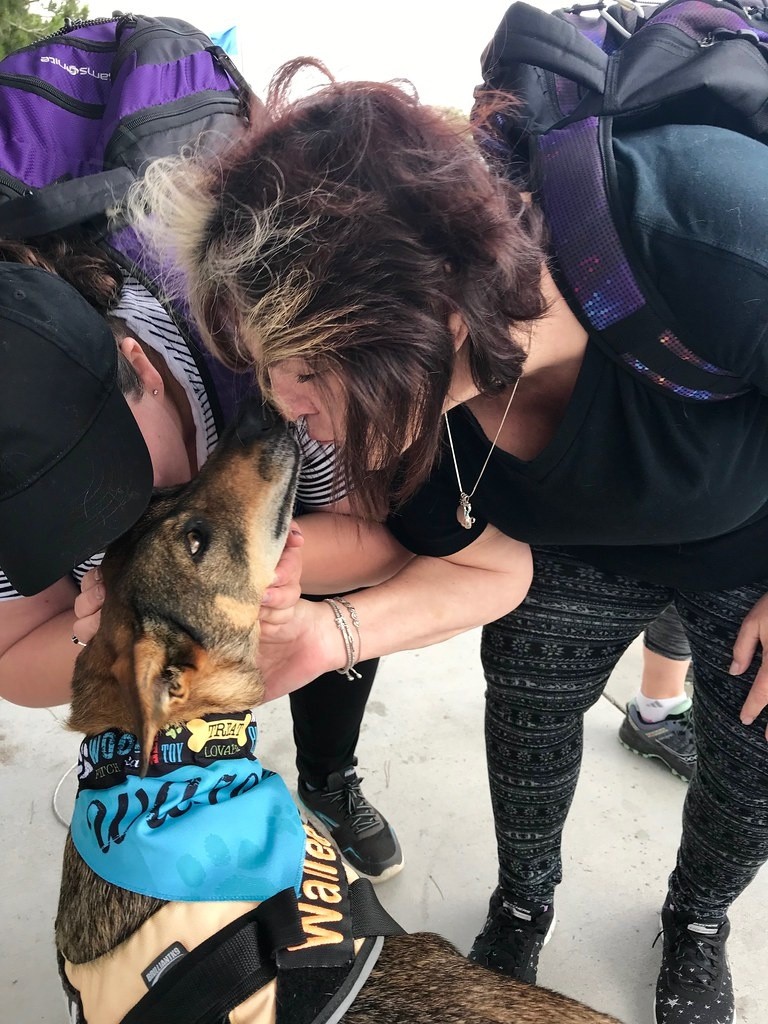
<box><xmin>0</xmin><ymin>12</ymin><xmax>274</xmax><ymax>440</ymax></box>
<box><xmin>470</xmin><ymin>0</ymin><xmax>768</xmax><ymax>399</ymax></box>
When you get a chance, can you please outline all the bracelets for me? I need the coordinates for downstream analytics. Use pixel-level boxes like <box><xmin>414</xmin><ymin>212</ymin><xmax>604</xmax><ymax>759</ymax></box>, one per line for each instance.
<box><xmin>322</xmin><ymin>598</ymin><xmax>363</xmax><ymax>681</ymax></box>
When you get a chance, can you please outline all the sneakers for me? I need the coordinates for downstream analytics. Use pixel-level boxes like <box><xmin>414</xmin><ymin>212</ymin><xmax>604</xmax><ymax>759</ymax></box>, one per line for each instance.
<box><xmin>618</xmin><ymin>699</ymin><xmax>697</xmax><ymax>782</ymax></box>
<box><xmin>654</xmin><ymin>893</ymin><xmax>732</xmax><ymax>1023</ymax></box>
<box><xmin>467</xmin><ymin>885</ymin><xmax>556</xmax><ymax>991</ymax></box>
<box><xmin>296</xmin><ymin>766</ymin><xmax>403</xmax><ymax>884</ymax></box>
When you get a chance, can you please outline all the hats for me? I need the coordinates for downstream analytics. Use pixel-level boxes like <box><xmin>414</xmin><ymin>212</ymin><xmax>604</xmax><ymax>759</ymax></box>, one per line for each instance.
<box><xmin>0</xmin><ymin>263</ymin><xmax>154</xmax><ymax>597</ymax></box>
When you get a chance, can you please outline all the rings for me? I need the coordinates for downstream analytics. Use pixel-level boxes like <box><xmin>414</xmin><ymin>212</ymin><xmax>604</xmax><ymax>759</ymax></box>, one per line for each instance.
<box><xmin>70</xmin><ymin>630</ymin><xmax>87</xmax><ymax>647</ymax></box>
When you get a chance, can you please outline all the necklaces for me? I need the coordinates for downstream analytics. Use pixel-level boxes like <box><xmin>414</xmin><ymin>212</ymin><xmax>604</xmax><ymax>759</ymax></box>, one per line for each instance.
<box><xmin>445</xmin><ymin>319</ymin><xmax>531</xmax><ymax>529</ymax></box>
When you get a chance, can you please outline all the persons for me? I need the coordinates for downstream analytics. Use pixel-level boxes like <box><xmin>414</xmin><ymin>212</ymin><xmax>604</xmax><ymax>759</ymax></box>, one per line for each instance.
<box><xmin>0</xmin><ymin>215</ymin><xmax>417</xmax><ymax>884</ymax></box>
<box><xmin>130</xmin><ymin>54</ymin><xmax>768</xmax><ymax>1024</ymax></box>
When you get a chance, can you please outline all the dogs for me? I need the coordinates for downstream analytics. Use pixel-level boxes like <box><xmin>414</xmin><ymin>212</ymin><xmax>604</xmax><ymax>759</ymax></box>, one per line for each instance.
<box><xmin>58</xmin><ymin>429</ymin><xmax>624</xmax><ymax>1024</ymax></box>
